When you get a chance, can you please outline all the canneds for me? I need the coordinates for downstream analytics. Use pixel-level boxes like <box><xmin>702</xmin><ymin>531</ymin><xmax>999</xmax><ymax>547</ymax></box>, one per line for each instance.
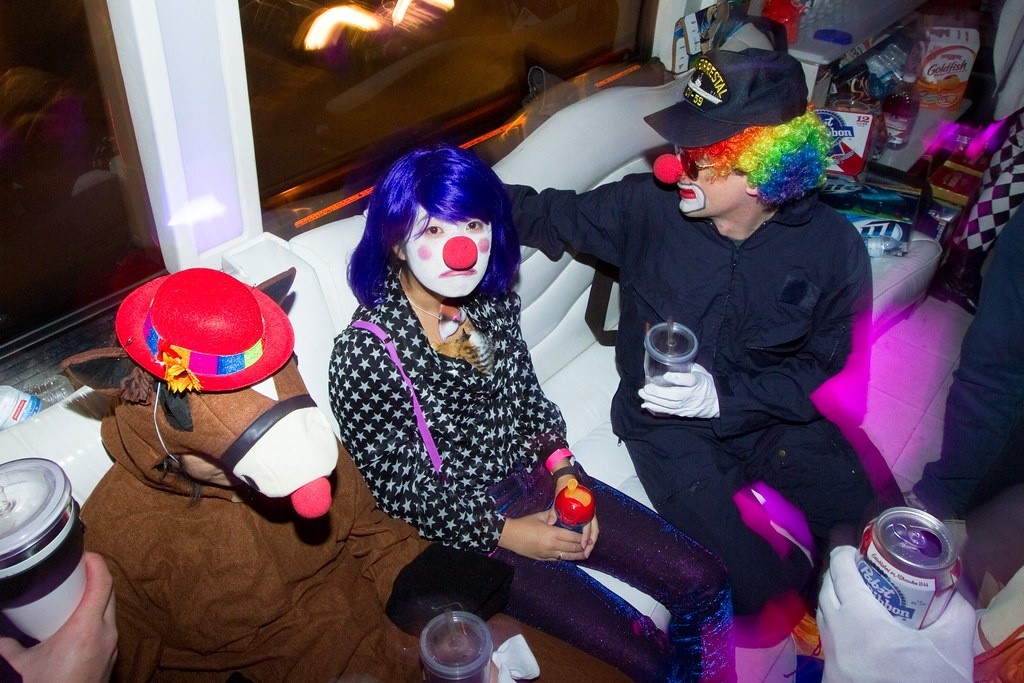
<box><xmin>855</xmin><ymin>507</ymin><xmax>962</xmax><ymax>630</ymax></box>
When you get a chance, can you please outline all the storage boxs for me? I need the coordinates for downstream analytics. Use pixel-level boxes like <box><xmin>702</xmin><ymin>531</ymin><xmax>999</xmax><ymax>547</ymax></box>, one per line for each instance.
<box><xmin>928</xmin><ymin>146</ymin><xmax>993</xmax><ymax>206</ymax></box>
<box><xmin>807</xmin><ymin>107</ymin><xmax>875</xmax><ymax>185</ymax></box>
<box><xmin>913</xmin><ymin>26</ymin><xmax>980</xmax><ymax>110</ymax></box>
<box><xmin>820</xmin><ymin>179</ymin><xmax>922</xmax><ymax>256</ymax></box>
<box><xmin>914</xmin><ymin>196</ymin><xmax>961</xmax><ymax>257</ymax></box>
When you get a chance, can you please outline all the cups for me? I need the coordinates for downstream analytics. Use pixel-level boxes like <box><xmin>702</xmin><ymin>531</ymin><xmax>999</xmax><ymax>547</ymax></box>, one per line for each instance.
<box><xmin>833</xmin><ymin>100</ymin><xmax>872</xmax><ymax>115</ymax></box>
<box><xmin>643</xmin><ymin>322</ymin><xmax>699</xmax><ymax>418</ymax></box>
<box><xmin>419</xmin><ymin>611</ymin><xmax>494</xmax><ymax>683</ymax></box>
<box><xmin>0</xmin><ymin>457</ymin><xmax>88</xmax><ymax>649</ymax></box>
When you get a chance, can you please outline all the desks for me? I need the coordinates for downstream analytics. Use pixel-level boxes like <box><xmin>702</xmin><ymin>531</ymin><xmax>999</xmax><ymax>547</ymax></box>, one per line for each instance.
<box><xmin>791</xmin><ymin>29</ymin><xmax>885</xmax><ymax>107</ymax></box>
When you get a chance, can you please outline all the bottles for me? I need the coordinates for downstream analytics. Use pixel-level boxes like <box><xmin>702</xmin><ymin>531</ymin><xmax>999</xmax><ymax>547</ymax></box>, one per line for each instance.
<box><xmin>861</xmin><ymin>234</ymin><xmax>908</xmax><ymax>258</ymax></box>
<box><xmin>880</xmin><ymin>73</ymin><xmax>920</xmax><ymax>151</ymax></box>
<box><xmin>20</xmin><ymin>375</ymin><xmax>76</xmax><ymax>405</ymax></box>
<box><xmin>0</xmin><ymin>384</ymin><xmax>53</xmax><ymax>431</ymax></box>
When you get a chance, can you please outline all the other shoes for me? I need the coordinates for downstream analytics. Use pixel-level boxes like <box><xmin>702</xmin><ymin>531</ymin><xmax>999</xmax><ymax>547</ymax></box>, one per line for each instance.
<box><xmin>903</xmin><ymin>488</ymin><xmax>928</xmax><ymax>513</ymax></box>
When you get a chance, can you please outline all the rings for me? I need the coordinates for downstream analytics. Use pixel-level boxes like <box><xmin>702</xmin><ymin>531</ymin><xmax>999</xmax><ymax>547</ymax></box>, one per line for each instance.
<box><xmin>557</xmin><ymin>550</ymin><xmax>563</xmax><ymax>561</ymax></box>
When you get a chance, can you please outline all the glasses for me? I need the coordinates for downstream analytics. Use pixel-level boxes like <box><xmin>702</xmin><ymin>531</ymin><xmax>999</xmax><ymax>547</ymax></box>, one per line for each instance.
<box><xmin>674</xmin><ymin>143</ymin><xmax>722</xmax><ymax>181</ymax></box>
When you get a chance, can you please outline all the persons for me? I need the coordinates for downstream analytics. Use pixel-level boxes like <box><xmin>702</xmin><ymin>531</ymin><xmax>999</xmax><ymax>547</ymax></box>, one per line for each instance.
<box><xmin>60</xmin><ymin>268</ymin><xmax>633</xmax><ymax>683</ymax></box>
<box><xmin>503</xmin><ymin>49</ymin><xmax>873</xmax><ymax>621</ymax></box>
<box><xmin>327</xmin><ymin>147</ymin><xmax>736</xmax><ymax>683</ymax></box>
<box><xmin>0</xmin><ymin>552</ymin><xmax>118</xmax><ymax>683</ymax></box>
<box><xmin>817</xmin><ymin>110</ymin><xmax>1024</xmax><ymax>683</ymax></box>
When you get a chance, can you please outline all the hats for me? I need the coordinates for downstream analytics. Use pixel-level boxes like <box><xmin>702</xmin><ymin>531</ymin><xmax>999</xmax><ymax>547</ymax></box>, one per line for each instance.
<box><xmin>643</xmin><ymin>48</ymin><xmax>809</xmax><ymax>147</ymax></box>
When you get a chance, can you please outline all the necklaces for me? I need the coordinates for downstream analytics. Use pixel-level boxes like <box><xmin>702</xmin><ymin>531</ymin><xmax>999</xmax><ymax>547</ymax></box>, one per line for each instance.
<box><xmin>405</xmin><ymin>295</ymin><xmax>467</xmax><ymax>341</ymax></box>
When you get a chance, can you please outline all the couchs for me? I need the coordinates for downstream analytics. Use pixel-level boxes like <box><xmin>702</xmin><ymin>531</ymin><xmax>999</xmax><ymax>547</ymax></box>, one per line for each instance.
<box><xmin>0</xmin><ymin>73</ymin><xmax>943</xmax><ymax>639</ymax></box>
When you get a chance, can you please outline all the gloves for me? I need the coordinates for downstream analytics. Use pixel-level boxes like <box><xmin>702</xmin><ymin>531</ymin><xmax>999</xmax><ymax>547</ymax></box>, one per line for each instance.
<box><xmin>816</xmin><ymin>545</ymin><xmax>977</xmax><ymax>683</ymax></box>
<box><xmin>638</xmin><ymin>362</ymin><xmax>720</xmax><ymax>420</ymax></box>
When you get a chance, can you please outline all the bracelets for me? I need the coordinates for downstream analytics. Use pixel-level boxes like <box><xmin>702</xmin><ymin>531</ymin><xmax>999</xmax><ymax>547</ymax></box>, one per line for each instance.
<box><xmin>553</xmin><ymin>466</ymin><xmax>584</xmax><ymax>487</ymax></box>
